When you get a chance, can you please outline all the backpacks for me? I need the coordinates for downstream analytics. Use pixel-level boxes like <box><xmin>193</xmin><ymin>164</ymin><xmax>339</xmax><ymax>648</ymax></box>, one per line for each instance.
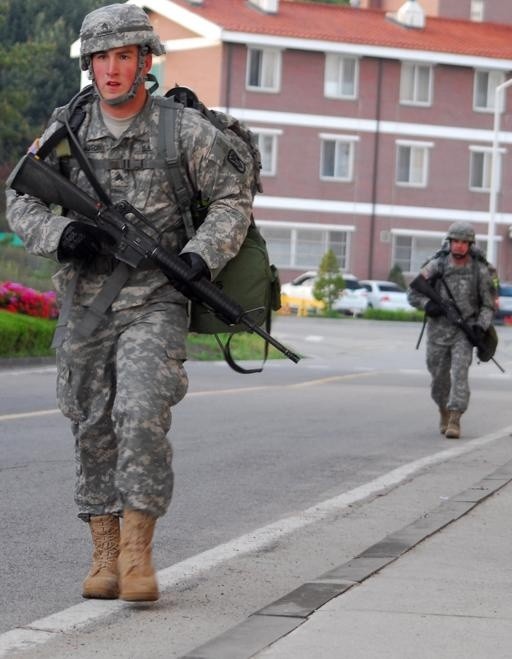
<box><xmin>158</xmin><ymin>85</ymin><xmax>284</xmax><ymax>335</ymax></box>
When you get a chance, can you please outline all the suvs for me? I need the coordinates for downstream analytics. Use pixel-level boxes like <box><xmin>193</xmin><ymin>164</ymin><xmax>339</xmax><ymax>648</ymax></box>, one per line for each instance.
<box><xmin>275</xmin><ymin>268</ymin><xmax>371</xmax><ymax>318</ymax></box>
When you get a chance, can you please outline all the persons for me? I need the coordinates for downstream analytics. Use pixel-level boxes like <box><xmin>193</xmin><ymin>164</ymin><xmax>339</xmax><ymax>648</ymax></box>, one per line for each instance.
<box><xmin>406</xmin><ymin>220</ymin><xmax>498</xmax><ymax>438</ymax></box>
<box><xmin>3</xmin><ymin>3</ymin><xmax>264</xmax><ymax>600</ymax></box>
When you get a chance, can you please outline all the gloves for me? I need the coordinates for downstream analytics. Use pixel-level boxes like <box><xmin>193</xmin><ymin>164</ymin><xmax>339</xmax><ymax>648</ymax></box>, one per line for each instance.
<box><xmin>164</xmin><ymin>252</ymin><xmax>210</xmax><ymax>302</ymax></box>
<box><xmin>59</xmin><ymin>219</ymin><xmax>121</xmax><ymax>262</ymax></box>
<box><xmin>424</xmin><ymin>301</ymin><xmax>446</xmax><ymax>317</ymax></box>
<box><xmin>467</xmin><ymin>325</ymin><xmax>484</xmax><ymax>345</ymax></box>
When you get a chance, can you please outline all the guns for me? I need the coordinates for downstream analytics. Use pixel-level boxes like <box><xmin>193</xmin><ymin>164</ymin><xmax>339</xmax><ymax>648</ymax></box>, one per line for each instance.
<box><xmin>409</xmin><ymin>275</ymin><xmax>505</xmax><ymax>373</ymax></box>
<box><xmin>10</xmin><ymin>153</ymin><xmax>299</xmax><ymax>363</ymax></box>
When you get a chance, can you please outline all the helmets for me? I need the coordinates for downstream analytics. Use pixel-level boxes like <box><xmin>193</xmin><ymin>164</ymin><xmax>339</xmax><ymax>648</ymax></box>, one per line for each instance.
<box><xmin>444</xmin><ymin>218</ymin><xmax>478</xmax><ymax>245</ymax></box>
<box><xmin>77</xmin><ymin>1</ymin><xmax>169</xmax><ymax>71</ymax></box>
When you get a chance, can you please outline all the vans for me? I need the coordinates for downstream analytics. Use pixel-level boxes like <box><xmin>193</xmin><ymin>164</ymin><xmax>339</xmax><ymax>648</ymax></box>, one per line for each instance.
<box><xmin>495</xmin><ymin>281</ymin><xmax>512</xmax><ymax>317</ymax></box>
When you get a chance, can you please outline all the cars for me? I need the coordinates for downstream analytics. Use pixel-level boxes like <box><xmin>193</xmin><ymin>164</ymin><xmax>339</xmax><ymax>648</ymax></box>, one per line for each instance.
<box><xmin>353</xmin><ymin>275</ymin><xmax>417</xmax><ymax>318</ymax></box>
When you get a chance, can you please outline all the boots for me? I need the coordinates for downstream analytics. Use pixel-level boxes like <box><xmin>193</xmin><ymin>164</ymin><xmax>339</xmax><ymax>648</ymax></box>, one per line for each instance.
<box><xmin>114</xmin><ymin>503</ymin><xmax>166</xmax><ymax>606</ymax></box>
<box><xmin>438</xmin><ymin>410</ymin><xmax>451</xmax><ymax>434</ymax></box>
<box><xmin>444</xmin><ymin>410</ymin><xmax>462</xmax><ymax>439</ymax></box>
<box><xmin>80</xmin><ymin>510</ymin><xmax>123</xmax><ymax>603</ymax></box>
